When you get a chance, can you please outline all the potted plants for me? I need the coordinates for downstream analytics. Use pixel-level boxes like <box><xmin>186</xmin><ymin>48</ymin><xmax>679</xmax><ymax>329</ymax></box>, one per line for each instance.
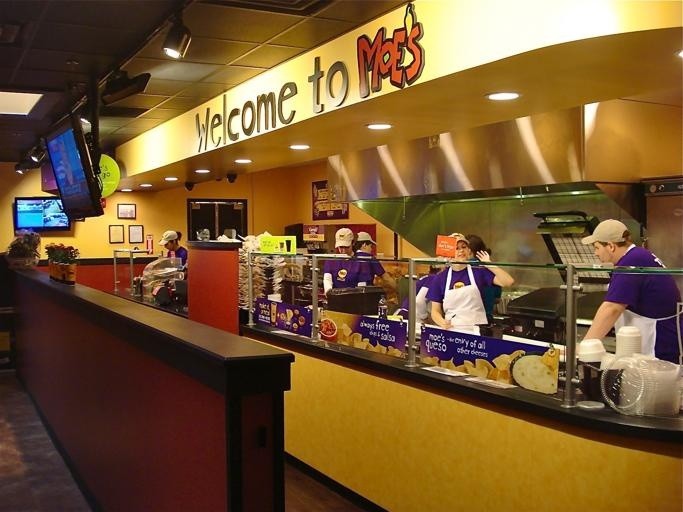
<box><xmin>46</xmin><ymin>242</ymin><xmax>79</xmax><ymax>285</ymax></box>
<box><xmin>6</xmin><ymin>232</ymin><xmax>42</xmax><ymax>271</ymax></box>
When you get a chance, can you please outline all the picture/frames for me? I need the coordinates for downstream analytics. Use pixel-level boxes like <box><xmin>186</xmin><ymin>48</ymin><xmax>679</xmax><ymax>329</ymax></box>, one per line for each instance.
<box><xmin>128</xmin><ymin>224</ymin><xmax>144</xmax><ymax>244</ymax></box>
<box><xmin>109</xmin><ymin>224</ymin><xmax>124</xmax><ymax>244</ymax></box>
<box><xmin>117</xmin><ymin>203</ymin><xmax>136</xmax><ymax>219</ymax></box>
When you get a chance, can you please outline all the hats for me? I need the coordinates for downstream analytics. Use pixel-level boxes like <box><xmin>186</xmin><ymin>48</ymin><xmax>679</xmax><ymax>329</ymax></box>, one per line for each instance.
<box><xmin>356</xmin><ymin>231</ymin><xmax>377</xmax><ymax>246</ymax></box>
<box><xmin>580</xmin><ymin>218</ymin><xmax>632</xmax><ymax>245</ymax></box>
<box><xmin>448</xmin><ymin>233</ymin><xmax>470</xmax><ymax>250</ymax></box>
<box><xmin>334</xmin><ymin>228</ymin><xmax>355</xmax><ymax>249</ymax></box>
<box><xmin>157</xmin><ymin>230</ymin><xmax>179</xmax><ymax>246</ymax></box>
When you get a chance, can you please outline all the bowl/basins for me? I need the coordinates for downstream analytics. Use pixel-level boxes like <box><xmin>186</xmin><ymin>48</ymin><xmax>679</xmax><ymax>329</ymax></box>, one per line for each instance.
<box><xmin>615</xmin><ymin>326</ymin><xmax>642</xmax><ymax>355</ymax></box>
<box><xmin>579</xmin><ymin>338</ymin><xmax>606</xmax><ymax>362</ymax></box>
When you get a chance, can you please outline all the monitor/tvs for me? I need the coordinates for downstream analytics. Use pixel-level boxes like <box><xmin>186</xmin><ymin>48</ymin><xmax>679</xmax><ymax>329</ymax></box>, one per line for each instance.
<box><xmin>15</xmin><ymin>197</ymin><xmax>71</xmax><ymax>230</ymax></box>
<box><xmin>44</xmin><ymin>113</ymin><xmax>105</xmax><ymax>219</ymax></box>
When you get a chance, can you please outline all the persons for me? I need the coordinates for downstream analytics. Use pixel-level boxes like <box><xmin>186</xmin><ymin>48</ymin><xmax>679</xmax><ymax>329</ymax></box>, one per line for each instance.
<box><xmin>465</xmin><ymin>235</ymin><xmax>502</xmax><ymax>322</ymax></box>
<box><xmin>560</xmin><ymin>220</ymin><xmax>683</xmax><ymax>364</ymax></box>
<box><xmin>352</xmin><ymin>232</ymin><xmax>395</xmax><ymax>289</ymax></box>
<box><xmin>426</xmin><ymin>234</ymin><xmax>515</xmax><ymax>336</ymax></box>
<box><xmin>323</xmin><ymin>228</ymin><xmax>372</xmax><ymax>299</ymax></box>
<box><xmin>159</xmin><ymin>230</ymin><xmax>188</xmax><ymax>265</ymax></box>
<box><xmin>57</xmin><ymin>143</ymin><xmax>81</xmax><ymax>190</ymax></box>
<box><xmin>393</xmin><ymin>264</ymin><xmax>446</xmax><ymax>340</ymax></box>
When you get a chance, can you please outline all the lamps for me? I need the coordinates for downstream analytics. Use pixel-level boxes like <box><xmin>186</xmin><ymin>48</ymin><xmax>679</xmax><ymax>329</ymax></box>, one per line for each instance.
<box><xmin>15</xmin><ymin>160</ymin><xmax>27</xmax><ymax>175</ymax></box>
<box><xmin>161</xmin><ymin>21</ymin><xmax>194</xmax><ymax>60</ymax></box>
<box><xmin>100</xmin><ymin>72</ymin><xmax>152</xmax><ymax>108</ymax></box>
<box><xmin>31</xmin><ymin>146</ymin><xmax>47</xmax><ymax>163</ymax></box>
